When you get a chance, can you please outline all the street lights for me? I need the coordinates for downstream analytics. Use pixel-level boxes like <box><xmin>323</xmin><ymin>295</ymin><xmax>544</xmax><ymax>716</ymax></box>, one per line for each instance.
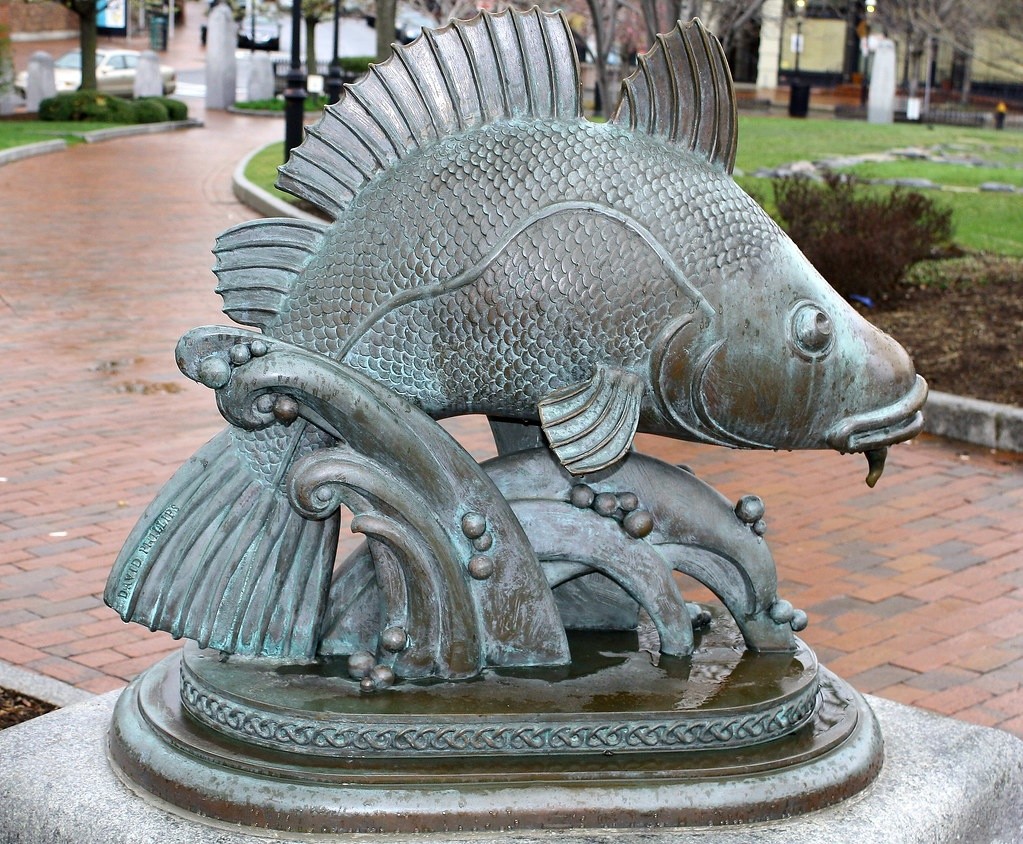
<box><xmin>792</xmin><ymin>1</ymin><xmax>807</xmax><ymax>76</ymax></box>
<box><xmin>860</xmin><ymin>0</ymin><xmax>876</xmax><ymax>106</ymax></box>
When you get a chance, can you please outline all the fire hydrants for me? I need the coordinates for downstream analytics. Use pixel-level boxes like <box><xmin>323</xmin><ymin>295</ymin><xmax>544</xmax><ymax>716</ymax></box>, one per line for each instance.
<box><xmin>994</xmin><ymin>101</ymin><xmax>1007</xmax><ymax>130</ymax></box>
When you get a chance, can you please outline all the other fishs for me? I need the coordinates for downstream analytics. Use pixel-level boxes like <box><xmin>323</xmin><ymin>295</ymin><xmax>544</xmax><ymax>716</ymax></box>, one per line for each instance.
<box><xmin>100</xmin><ymin>9</ymin><xmax>929</xmax><ymax>658</ymax></box>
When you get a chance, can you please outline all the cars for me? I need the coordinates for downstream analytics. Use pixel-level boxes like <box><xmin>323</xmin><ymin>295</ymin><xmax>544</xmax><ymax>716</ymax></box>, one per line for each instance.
<box><xmin>237</xmin><ymin>15</ymin><xmax>284</xmax><ymax>53</ymax></box>
<box><xmin>12</xmin><ymin>48</ymin><xmax>176</xmax><ymax>107</ymax></box>
<box><xmin>394</xmin><ymin>13</ymin><xmax>437</xmax><ymax>47</ymax></box>
